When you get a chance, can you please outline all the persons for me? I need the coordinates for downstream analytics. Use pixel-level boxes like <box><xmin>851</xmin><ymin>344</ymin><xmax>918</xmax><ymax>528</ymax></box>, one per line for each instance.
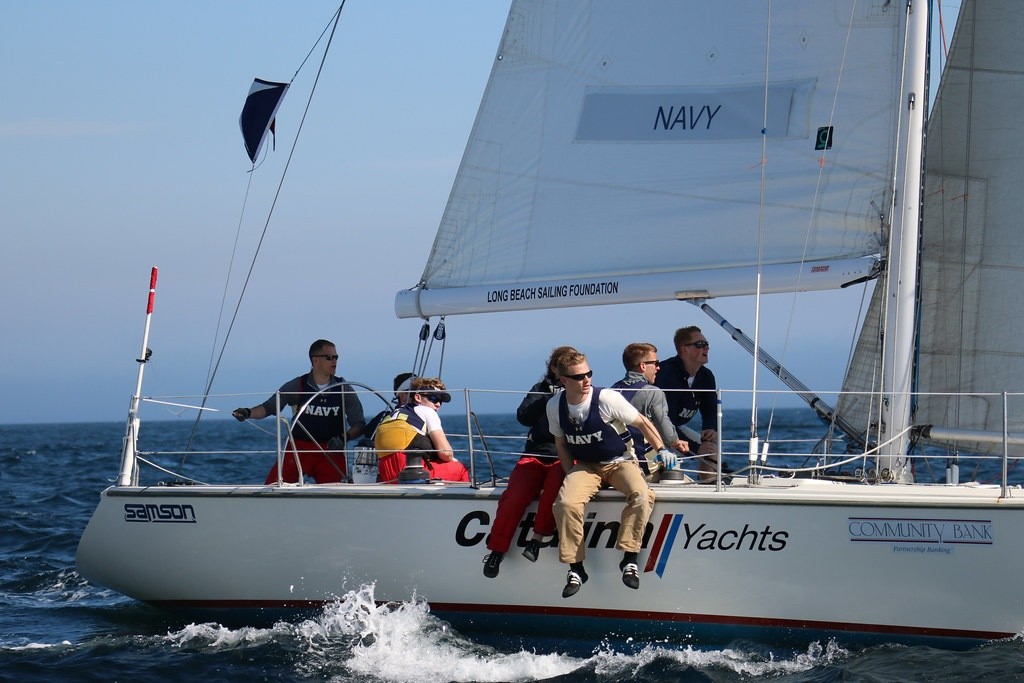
<box><xmin>379</xmin><ymin>378</ymin><xmax>471</xmax><ymax>482</ymax></box>
<box><xmin>546</xmin><ymin>354</ymin><xmax>678</xmax><ymax>599</ymax></box>
<box><xmin>365</xmin><ymin>372</ymin><xmax>416</xmax><ymax>439</ymax></box>
<box><xmin>483</xmin><ymin>346</ymin><xmax>576</xmax><ymax>579</ymax></box>
<box><xmin>611</xmin><ymin>344</ymin><xmax>689</xmax><ymax>481</ymax></box>
<box><xmin>232</xmin><ymin>340</ymin><xmax>366</xmax><ymax>483</ymax></box>
<box><xmin>653</xmin><ymin>326</ymin><xmax>718</xmax><ymax>485</ymax></box>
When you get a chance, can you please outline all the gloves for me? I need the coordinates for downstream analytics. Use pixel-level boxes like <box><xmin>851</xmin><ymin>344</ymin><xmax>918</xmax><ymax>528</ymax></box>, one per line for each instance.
<box><xmin>654</xmin><ymin>450</ymin><xmax>678</xmax><ymax>471</ymax></box>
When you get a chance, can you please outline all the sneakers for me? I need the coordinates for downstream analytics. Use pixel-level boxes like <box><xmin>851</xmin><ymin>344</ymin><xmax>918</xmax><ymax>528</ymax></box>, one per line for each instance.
<box><xmin>522</xmin><ymin>538</ymin><xmax>541</xmax><ymax>562</ymax></box>
<box><xmin>482</xmin><ymin>550</ymin><xmax>505</xmax><ymax>578</ymax></box>
<box><xmin>562</xmin><ymin>566</ymin><xmax>588</xmax><ymax>598</ymax></box>
<box><xmin>619</xmin><ymin>559</ymin><xmax>640</xmax><ymax>589</ymax></box>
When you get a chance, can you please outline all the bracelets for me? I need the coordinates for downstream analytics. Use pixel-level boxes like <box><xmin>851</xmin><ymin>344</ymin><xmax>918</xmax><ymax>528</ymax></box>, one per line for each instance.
<box><xmin>657</xmin><ymin>447</ymin><xmax>666</xmax><ymax>452</ymax></box>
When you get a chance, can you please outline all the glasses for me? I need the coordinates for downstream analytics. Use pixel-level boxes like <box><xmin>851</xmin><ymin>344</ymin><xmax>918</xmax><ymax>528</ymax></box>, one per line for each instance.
<box><xmin>683</xmin><ymin>340</ymin><xmax>708</xmax><ymax>348</ymax></box>
<box><xmin>420</xmin><ymin>394</ymin><xmax>442</xmax><ymax>405</ymax></box>
<box><xmin>311</xmin><ymin>354</ymin><xmax>338</xmax><ymax>361</ymax></box>
<box><xmin>636</xmin><ymin>360</ymin><xmax>660</xmax><ymax>367</ymax></box>
<box><xmin>561</xmin><ymin>370</ymin><xmax>593</xmax><ymax>380</ymax></box>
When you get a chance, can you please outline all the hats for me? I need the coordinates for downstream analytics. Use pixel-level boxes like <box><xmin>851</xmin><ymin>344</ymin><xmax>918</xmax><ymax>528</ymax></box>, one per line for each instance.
<box><xmin>418</xmin><ymin>386</ymin><xmax>451</xmax><ymax>403</ymax></box>
<box><xmin>395</xmin><ymin>376</ymin><xmax>417</xmax><ymax>398</ymax></box>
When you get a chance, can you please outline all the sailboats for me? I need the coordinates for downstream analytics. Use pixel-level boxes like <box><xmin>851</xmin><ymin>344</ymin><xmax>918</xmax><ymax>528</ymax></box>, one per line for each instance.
<box><xmin>74</xmin><ymin>0</ymin><xmax>1020</xmax><ymax>649</ymax></box>
